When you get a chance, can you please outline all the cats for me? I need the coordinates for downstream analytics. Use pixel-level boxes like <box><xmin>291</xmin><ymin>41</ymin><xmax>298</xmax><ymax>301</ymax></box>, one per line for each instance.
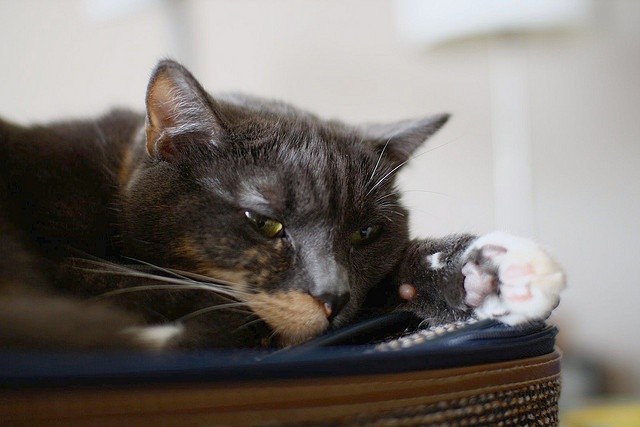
<box><xmin>0</xmin><ymin>55</ymin><xmax>572</xmax><ymax>351</ymax></box>
